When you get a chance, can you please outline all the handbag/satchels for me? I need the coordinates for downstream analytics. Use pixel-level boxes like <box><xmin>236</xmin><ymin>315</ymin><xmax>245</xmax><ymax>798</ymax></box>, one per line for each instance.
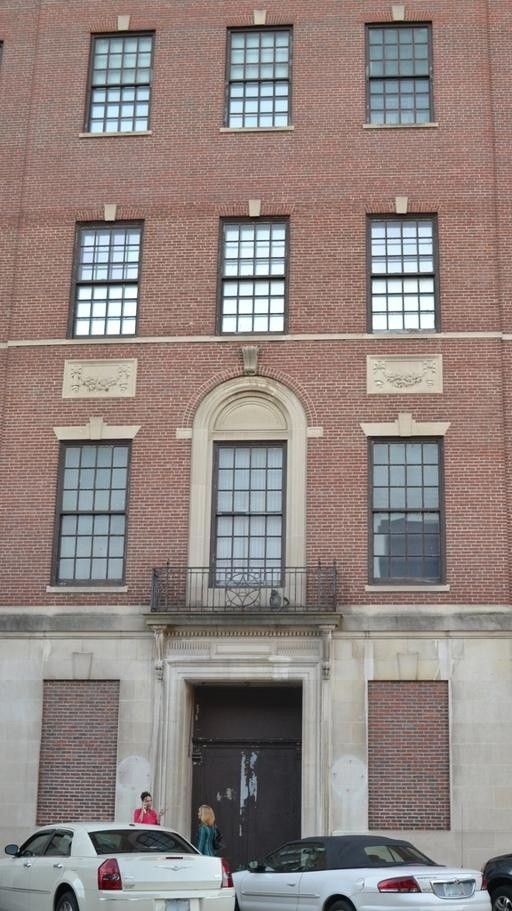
<box><xmin>214</xmin><ymin>829</ymin><xmax>223</xmax><ymax>849</ymax></box>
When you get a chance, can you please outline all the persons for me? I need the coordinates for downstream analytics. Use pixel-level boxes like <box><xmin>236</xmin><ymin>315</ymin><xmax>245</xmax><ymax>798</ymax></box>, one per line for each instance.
<box><xmin>133</xmin><ymin>792</ymin><xmax>167</xmax><ymax>827</ymax></box>
<box><xmin>195</xmin><ymin>804</ymin><xmax>220</xmax><ymax>857</ymax></box>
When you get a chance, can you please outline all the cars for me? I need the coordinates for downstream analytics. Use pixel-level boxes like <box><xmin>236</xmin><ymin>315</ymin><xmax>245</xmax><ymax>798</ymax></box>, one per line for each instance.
<box><xmin>481</xmin><ymin>853</ymin><xmax>512</xmax><ymax>911</ymax></box>
<box><xmin>1</xmin><ymin>821</ymin><xmax>235</xmax><ymax>911</ymax></box>
<box><xmin>231</xmin><ymin>834</ymin><xmax>493</xmax><ymax>911</ymax></box>
